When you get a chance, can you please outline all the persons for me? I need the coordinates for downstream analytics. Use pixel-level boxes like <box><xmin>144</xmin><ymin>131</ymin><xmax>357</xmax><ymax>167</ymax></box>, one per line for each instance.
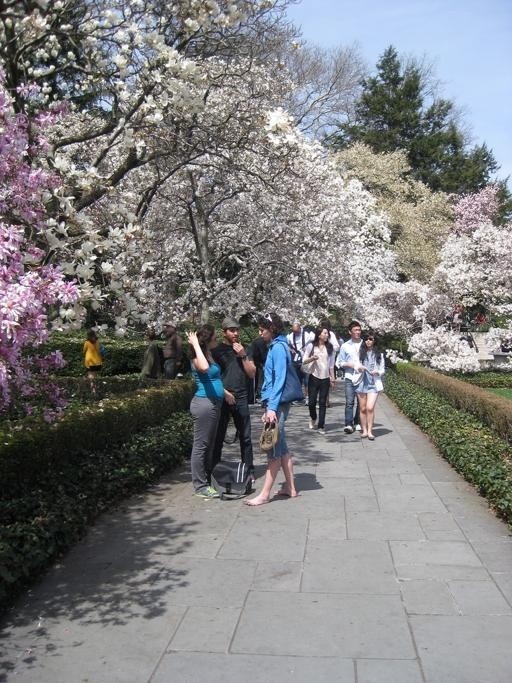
<box><xmin>206</xmin><ymin>317</ymin><xmax>255</xmax><ymax>483</ymax></box>
<box><xmin>353</xmin><ymin>329</ymin><xmax>386</xmax><ymax>440</ymax></box>
<box><xmin>242</xmin><ymin>312</ymin><xmax>305</xmax><ymax>506</ymax></box>
<box><xmin>335</xmin><ymin>321</ymin><xmax>364</xmax><ymax>433</ymax></box>
<box><xmin>81</xmin><ymin>330</ymin><xmax>103</xmax><ymax>398</ymax></box>
<box><xmin>141</xmin><ymin>327</ymin><xmax>160</xmax><ymax>389</ymax></box>
<box><xmin>163</xmin><ymin>323</ymin><xmax>182</xmax><ymax>378</ymax></box>
<box><xmin>185</xmin><ymin>323</ymin><xmax>225</xmax><ymax>498</ymax></box>
<box><xmin>285</xmin><ymin>323</ymin><xmax>345</xmax><ymax>406</ymax></box>
<box><xmin>302</xmin><ymin>325</ymin><xmax>336</xmax><ymax>435</ymax></box>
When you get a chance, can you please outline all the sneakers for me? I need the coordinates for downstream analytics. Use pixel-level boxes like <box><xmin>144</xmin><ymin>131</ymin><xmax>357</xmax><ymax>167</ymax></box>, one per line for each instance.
<box><xmin>259</xmin><ymin>421</ymin><xmax>278</xmax><ymax>450</ymax></box>
<box><xmin>306</xmin><ymin>396</ymin><xmax>374</xmax><ymax>440</ymax></box>
<box><xmin>196</xmin><ymin>486</ymin><xmax>219</xmax><ymax>498</ymax></box>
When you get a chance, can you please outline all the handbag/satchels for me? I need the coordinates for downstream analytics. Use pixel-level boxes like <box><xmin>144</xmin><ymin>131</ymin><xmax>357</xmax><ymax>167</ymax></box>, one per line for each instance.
<box><xmin>271</xmin><ymin>365</ymin><xmax>306</xmax><ymax>406</ymax></box>
<box><xmin>300</xmin><ymin>354</ymin><xmax>314</xmax><ymax>374</ymax></box>
<box><xmin>211</xmin><ymin>461</ymin><xmax>252</xmax><ymax>501</ymax></box>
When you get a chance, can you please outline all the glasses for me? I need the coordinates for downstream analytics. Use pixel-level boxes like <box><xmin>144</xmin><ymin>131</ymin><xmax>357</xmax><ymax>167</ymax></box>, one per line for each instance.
<box><xmin>363</xmin><ymin>338</ymin><xmax>374</xmax><ymax>341</ymax></box>
<box><xmin>264</xmin><ymin>312</ymin><xmax>272</xmax><ymax>323</ymax></box>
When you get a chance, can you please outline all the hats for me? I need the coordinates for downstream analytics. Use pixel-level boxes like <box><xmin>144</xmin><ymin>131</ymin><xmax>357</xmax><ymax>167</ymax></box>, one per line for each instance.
<box><xmin>221</xmin><ymin>316</ymin><xmax>241</xmax><ymax>330</ymax></box>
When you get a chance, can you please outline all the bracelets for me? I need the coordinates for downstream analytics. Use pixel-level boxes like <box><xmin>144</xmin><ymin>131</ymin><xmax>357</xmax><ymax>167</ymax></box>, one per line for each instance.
<box><xmin>240</xmin><ymin>354</ymin><xmax>247</xmax><ymax>360</ymax></box>
